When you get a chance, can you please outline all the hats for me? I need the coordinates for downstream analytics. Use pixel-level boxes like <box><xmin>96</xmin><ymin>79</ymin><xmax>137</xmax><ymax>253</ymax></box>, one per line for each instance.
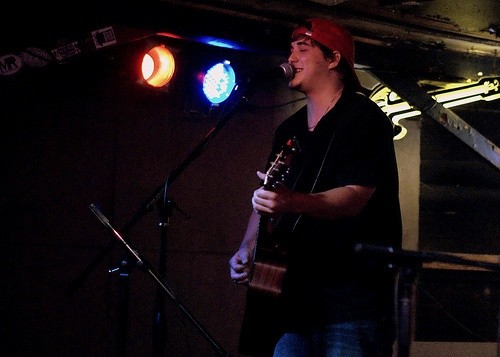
<box><xmin>292</xmin><ymin>16</ymin><xmax>358</xmax><ymax>81</ymax></box>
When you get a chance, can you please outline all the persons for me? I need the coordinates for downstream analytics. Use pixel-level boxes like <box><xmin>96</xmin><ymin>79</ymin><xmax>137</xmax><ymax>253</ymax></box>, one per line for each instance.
<box><xmin>229</xmin><ymin>17</ymin><xmax>404</xmax><ymax>357</ymax></box>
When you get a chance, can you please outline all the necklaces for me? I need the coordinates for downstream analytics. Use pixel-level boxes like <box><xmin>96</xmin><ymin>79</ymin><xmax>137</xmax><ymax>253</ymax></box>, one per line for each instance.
<box><xmin>307</xmin><ymin>88</ymin><xmax>343</xmax><ymax>128</ymax></box>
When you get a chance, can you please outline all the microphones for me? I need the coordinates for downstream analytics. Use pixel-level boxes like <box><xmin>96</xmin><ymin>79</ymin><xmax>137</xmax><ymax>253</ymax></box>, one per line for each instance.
<box><xmin>239</xmin><ymin>63</ymin><xmax>294</xmax><ymax>86</ymax></box>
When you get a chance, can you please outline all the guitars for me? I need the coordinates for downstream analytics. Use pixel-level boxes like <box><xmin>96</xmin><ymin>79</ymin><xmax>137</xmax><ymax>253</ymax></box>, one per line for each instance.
<box><xmin>368</xmin><ymin>76</ymin><xmax>500</xmax><ymax>141</ymax></box>
<box><xmin>247</xmin><ymin>136</ymin><xmax>298</xmax><ymax>295</ymax></box>
